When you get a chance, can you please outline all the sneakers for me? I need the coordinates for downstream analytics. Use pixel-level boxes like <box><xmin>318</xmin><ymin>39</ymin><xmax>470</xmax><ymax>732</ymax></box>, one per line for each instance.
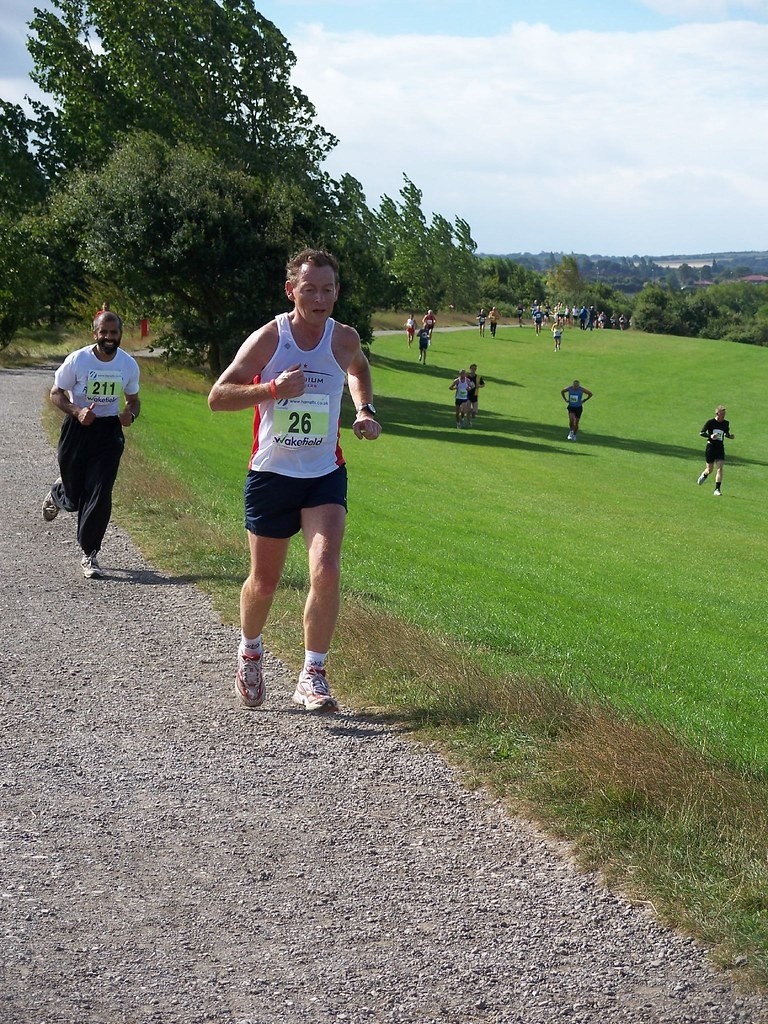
<box><xmin>457</xmin><ymin>422</ymin><xmax>461</xmax><ymax>429</ymax></box>
<box><xmin>41</xmin><ymin>476</ymin><xmax>62</xmax><ymax>521</ymax></box>
<box><xmin>697</xmin><ymin>471</ymin><xmax>709</xmax><ymax>485</ymax></box>
<box><xmin>292</xmin><ymin>667</ymin><xmax>338</xmax><ymax>714</ymax></box>
<box><xmin>558</xmin><ymin>346</ymin><xmax>561</xmax><ymax>350</ymax></box>
<box><xmin>418</xmin><ymin>356</ymin><xmax>422</xmax><ymax>361</ymax></box>
<box><xmin>234</xmin><ymin>644</ymin><xmax>265</xmax><ymax>707</ymax></box>
<box><xmin>461</xmin><ymin>420</ymin><xmax>464</xmax><ymax>427</ymax></box>
<box><xmin>572</xmin><ymin>435</ymin><xmax>576</xmax><ymax>440</ymax></box>
<box><xmin>468</xmin><ymin>421</ymin><xmax>472</xmax><ymax>426</ymax></box>
<box><xmin>555</xmin><ymin>348</ymin><xmax>558</xmax><ymax>352</ymax></box>
<box><xmin>714</xmin><ymin>489</ymin><xmax>722</xmax><ymax>496</ymax></box>
<box><xmin>568</xmin><ymin>430</ymin><xmax>574</xmax><ymax>439</ymax></box>
<box><xmin>81</xmin><ymin>550</ymin><xmax>104</xmax><ymax>579</ymax></box>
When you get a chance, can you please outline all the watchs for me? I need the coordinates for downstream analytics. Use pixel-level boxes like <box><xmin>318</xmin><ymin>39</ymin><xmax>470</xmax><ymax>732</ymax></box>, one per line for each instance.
<box><xmin>132</xmin><ymin>412</ymin><xmax>137</xmax><ymax>423</ymax></box>
<box><xmin>358</xmin><ymin>403</ymin><xmax>377</xmax><ymax>415</ymax></box>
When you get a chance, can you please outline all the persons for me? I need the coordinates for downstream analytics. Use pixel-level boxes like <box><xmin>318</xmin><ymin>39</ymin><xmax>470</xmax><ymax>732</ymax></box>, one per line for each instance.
<box><xmin>698</xmin><ymin>404</ymin><xmax>735</xmax><ymax>495</ymax></box>
<box><xmin>455</xmin><ymin>364</ymin><xmax>485</xmax><ymax>425</ymax></box>
<box><xmin>530</xmin><ymin>299</ymin><xmax>635</xmax><ymax>332</ymax></box>
<box><xmin>404</xmin><ymin>314</ymin><xmax>417</xmax><ymax>348</ymax></box>
<box><xmin>488</xmin><ymin>307</ymin><xmax>498</xmax><ymax>338</ymax></box>
<box><xmin>561</xmin><ymin>379</ymin><xmax>593</xmax><ymax>441</ymax></box>
<box><xmin>94</xmin><ymin>300</ymin><xmax>110</xmax><ymax>320</ymax></box>
<box><xmin>421</xmin><ymin>309</ymin><xmax>436</xmax><ymax>346</ymax></box>
<box><xmin>476</xmin><ymin>308</ymin><xmax>487</xmax><ymax>338</ymax></box>
<box><xmin>207</xmin><ymin>249</ymin><xmax>384</xmax><ymax>712</ymax></box>
<box><xmin>41</xmin><ymin>313</ymin><xmax>141</xmax><ymax>578</ymax></box>
<box><xmin>417</xmin><ymin>323</ymin><xmax>430</xmax><ymax>364</ymax></box>
<box><xmin>533</xmin><ymin>306</ymin><xmax>543</xmax><ymax>336</ymax></box>
<box><xmin>516</xmin><ymin>303</ymin><xmax>526</xmax><ymax>328</ymax></box>
<box><xmin>449</xmin><ymin>368</ymin><xmax>472</xmax><ymax>429</ymax></box>
<box><xmin>551</xmin><ymin>320</ymin><xmax>564</xmax><ymax>352</ymax></box>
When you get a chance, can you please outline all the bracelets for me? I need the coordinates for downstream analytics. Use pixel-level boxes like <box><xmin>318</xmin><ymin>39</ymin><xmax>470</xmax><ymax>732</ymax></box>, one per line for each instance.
<box><xmin>270</xmin><ymin>378</ymin><xmax>279</xmax><ymax>401</ymax></box>
